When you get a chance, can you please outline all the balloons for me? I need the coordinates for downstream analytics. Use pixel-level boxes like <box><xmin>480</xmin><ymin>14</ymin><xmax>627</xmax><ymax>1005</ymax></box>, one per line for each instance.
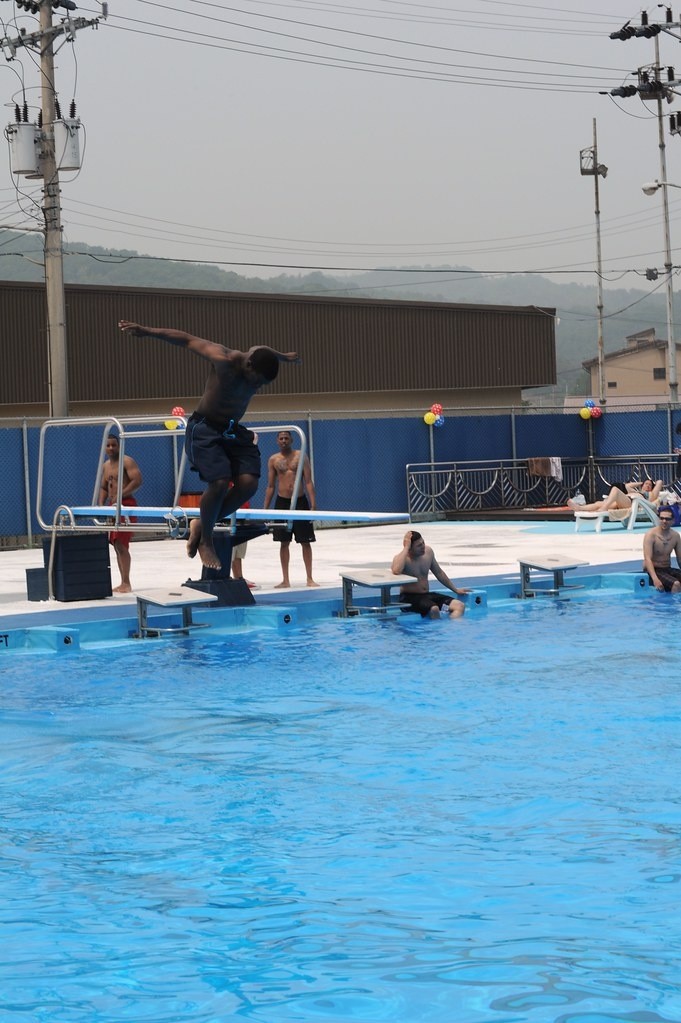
<box><xmin>424</xmin><ymin>404</ymin><xmax>444</xmax><ymax>427</ymax></box>
<box><xmin>165</xmin><ymin>407</ymin><xmax>188</xmax><ymax>429</ymax></box>
<box><xmin>580</xmin><ymin>400</ymin><xmax>602</xmax><ymax>420</ymax></box>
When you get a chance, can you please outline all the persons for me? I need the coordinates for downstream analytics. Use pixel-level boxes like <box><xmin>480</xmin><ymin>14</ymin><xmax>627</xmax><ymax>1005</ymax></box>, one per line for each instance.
<box><xmin>567</xmin><ymin>479</ymin><xmax>663</xmax><ymax>512</ymax></box>
<box><xmin>392</xmin><ymin>530</ymin><xmax>474</xmax><ymax>620</ymax></box>
<box><xmin>643</xmin><ymin>508</ymin><xmax>681</xmax><ymax>594</ymax></box>
<box><xmin>119</xmin><ymin>320</ymin><xmax>303</xmax><ymax>571</ymax></box>
<box><xmin>262</xmin><ymin>431</ymin><xmax>320</xmax><ymax>588</ymax></box>
<box><xmin>229</xmin><ymin>480</ymin><xmax>261</xmax><ymax>590</ymax></box>
<box><xmin>98</xmin><ymin>434</ymin><xmax>142</xmax><ymax>592</ymax></box>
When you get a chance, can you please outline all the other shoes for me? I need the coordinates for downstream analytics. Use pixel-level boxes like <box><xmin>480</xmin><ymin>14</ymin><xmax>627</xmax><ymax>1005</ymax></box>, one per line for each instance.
<box><xmin>245</xmin><ymin>579</ymin><xmax>261</xmax><ymax>590</ymax></box>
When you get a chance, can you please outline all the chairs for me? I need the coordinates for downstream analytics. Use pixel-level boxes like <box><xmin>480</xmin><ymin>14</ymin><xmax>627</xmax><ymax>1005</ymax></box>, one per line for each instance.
<box><xmin>575</xmin><ymin>489</ymin><xmax>668</xmax><ymax>532</ymax></box>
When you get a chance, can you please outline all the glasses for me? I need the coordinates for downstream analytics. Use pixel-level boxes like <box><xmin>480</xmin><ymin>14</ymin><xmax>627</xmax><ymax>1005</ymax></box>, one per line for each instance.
<box><xmin>660</xmin><ymin>517</ymin><xmax>673</xmax><ymax>521</ymax></box>
